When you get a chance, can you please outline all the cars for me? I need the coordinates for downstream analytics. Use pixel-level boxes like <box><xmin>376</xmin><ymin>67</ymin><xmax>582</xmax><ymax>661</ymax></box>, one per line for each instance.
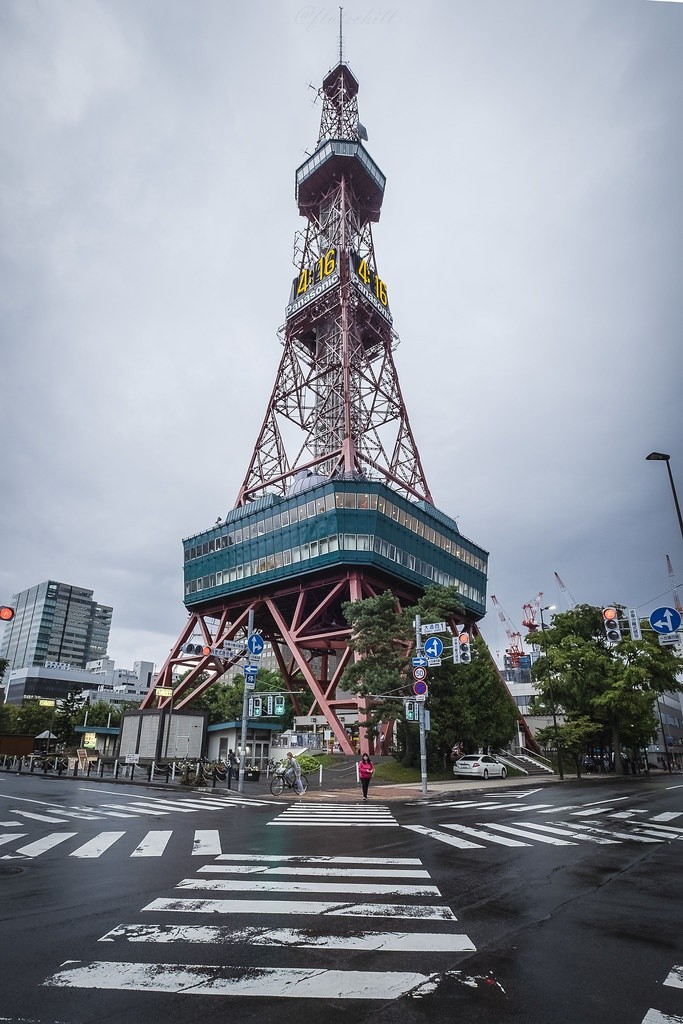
<box><xmin>452</xmin><ymin>753</ymin><xmax>508</xmax><ymax>780</ymax></box>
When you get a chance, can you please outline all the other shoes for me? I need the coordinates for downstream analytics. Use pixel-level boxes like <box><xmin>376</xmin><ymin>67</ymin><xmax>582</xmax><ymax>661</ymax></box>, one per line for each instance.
<box><xmin>299</xmin><ymin>792</ymin><xmax>306</xmax><ymax>795</ymax></box>
<box><xmin>363</xmin><ymin>797</ymin><xmax>367</xmax><ymax>800</ymax></box>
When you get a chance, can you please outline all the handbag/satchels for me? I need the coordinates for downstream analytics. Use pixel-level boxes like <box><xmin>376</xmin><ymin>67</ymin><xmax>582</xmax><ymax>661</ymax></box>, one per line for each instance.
<box><xmin>235</xmin><ymin>757</ymin><xmax>240</xmax><ymax>763</ymax></box>
<box><xmin>276</xmin><ymin>765</ymin><xmax>286</xmax><ymax>774</ymax></box>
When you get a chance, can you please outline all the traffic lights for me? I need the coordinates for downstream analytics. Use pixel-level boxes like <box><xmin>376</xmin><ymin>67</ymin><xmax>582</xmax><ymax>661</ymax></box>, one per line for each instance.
<box><xmin>406</xmin><ymin>702</ymin><xmax>414</xmax><ymax>721</ymax></box>
<box><xmin>458</xmin><ymin>632</ymin><xmax>472</xmax><ymax>664</ymax></box>
<box><xmin>0</xmin><ymin>605</ymin><xmax>15</xmax><ymax>621</ymax></box>
<box><xmin>602</xmin><ymin>607</ymin><xmax>622</xmax><ymax>643</ymax></box>
<box><xmin>253</xmin><ymin>698</ymin><xmax>263</xmax><ymax>716</ymax></box>
<box><xmin>180</xmin><ymin>643</ymin><xmax>211</xmax><ymax>656</ymax></box>
<box><xmin>274</xmin><ymin>695</ymin><xmax>286</xmax><ymax>716</ymax></box>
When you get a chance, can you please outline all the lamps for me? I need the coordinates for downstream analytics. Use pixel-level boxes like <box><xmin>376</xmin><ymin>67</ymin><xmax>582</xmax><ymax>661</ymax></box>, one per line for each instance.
<box><xmin>215</xmin><ymin>517</ymin><xmax>222</xmax><ymax>524</ymax></box>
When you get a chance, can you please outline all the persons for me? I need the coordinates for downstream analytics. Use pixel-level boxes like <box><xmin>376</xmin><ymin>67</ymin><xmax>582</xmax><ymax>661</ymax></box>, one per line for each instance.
<box><xmin>228</xmin><ymin>749</ymin><xmax>236</xmax><ymax>779</ymax></box>
<box><xmin>581</xmin><ymin>752</ymin><xmax>609</xmax><ymax>774</ymax></box>
<box><xmin>358</xmin><ymin>753</ymin><xmax>374</xmax><ymax>800</ymax></box>
<box><xmin>663</xmin><ymin>760</ymin><xmax>682</xmax><ymax>771</ymax></box>
<box><xmin>380</xmin><ymin>732</ymin><xmax>386</xmax><ymax>756</ymax></box>
<box><xmin>286</xmin><ymin>752</ymin><xmax>305</xmax><ymax>795</ymax></box>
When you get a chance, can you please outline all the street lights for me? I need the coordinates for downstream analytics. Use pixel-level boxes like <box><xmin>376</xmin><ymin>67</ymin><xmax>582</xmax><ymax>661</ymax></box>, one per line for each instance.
<box><xmin>645</xmin><ymin>451</ymin><xmax>683</xmax><ymax>542</ymax></box>
<box><xmin>539</xmin><ymin>604</ymin><xmax>564</xmax><ymax>780</ymax></box>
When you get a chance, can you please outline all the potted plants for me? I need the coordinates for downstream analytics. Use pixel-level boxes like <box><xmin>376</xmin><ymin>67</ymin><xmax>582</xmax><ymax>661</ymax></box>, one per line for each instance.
<box><xmin>244</xmin><ymin>766</ymin><xmax>261</xmax><ymax>781</ymax></box>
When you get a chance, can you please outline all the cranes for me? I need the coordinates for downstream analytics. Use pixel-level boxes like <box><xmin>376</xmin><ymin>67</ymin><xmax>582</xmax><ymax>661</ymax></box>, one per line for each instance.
<box><xmin>521</xmin><ymin>590</ymin><xmax>544</xmax><ymax>652</ymax></box>
<box><xmin>491</xmin><ymin>594</ymin><xmax>525</xmax><ymax>669</ymax></box>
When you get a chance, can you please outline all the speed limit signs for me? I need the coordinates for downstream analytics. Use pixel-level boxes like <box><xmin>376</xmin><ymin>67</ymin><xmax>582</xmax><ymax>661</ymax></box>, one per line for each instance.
<box><xmin>414</xmin><ymin>666</ymin><xmax>426</xmax><ymax>681</ymax></box>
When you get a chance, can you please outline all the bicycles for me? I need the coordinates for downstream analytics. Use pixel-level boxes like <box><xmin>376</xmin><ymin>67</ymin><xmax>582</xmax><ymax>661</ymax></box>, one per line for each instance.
<box><xmin>269</xmin><ymin>767</ymin><xmax>308</xmax><ymax>796</ymax></box>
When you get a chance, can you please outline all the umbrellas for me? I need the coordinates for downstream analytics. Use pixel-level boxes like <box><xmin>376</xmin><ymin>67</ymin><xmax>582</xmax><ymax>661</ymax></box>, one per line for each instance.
<box><xmin>35</xmin><ymin>730</ymin><xmax>57</xmax><ymax>739</ymax></box>
